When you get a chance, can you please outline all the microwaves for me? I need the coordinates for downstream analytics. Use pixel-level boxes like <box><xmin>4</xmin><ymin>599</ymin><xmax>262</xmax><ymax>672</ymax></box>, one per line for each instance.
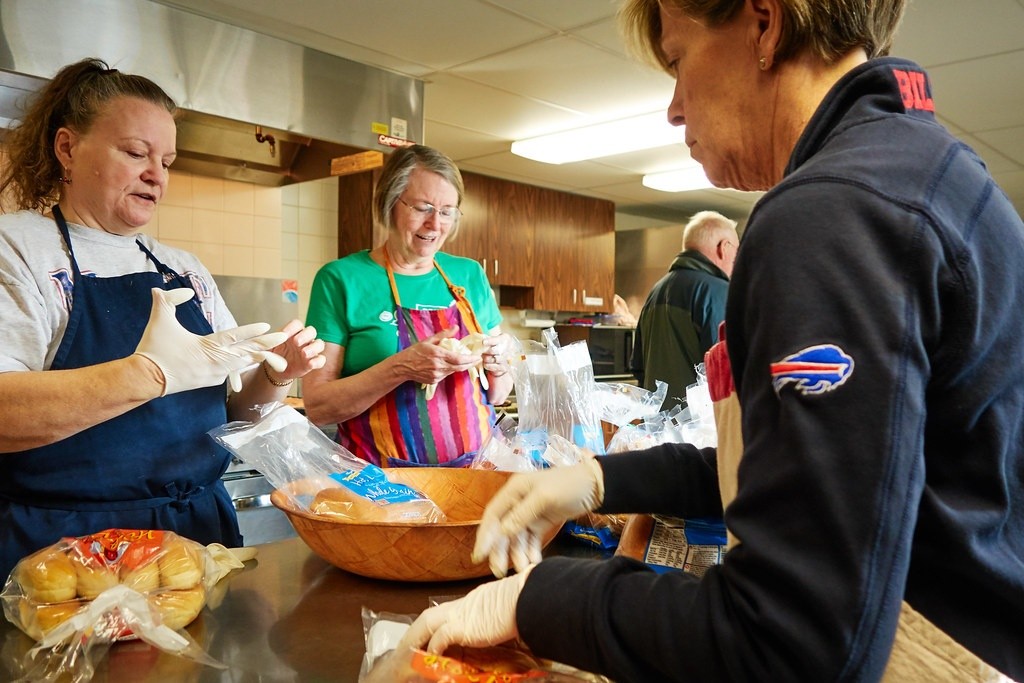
<box><xmin>553</xmin><ymin>320</ymin><xmax>640</xmax><ymax>382</ymax></box>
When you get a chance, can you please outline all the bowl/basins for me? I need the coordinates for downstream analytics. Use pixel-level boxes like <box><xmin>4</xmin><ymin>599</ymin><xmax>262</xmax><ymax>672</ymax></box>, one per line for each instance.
<box><xmin>269</xmin><ymin>466</ymin><xmax>569</xmax><ymax>584</ymax></box>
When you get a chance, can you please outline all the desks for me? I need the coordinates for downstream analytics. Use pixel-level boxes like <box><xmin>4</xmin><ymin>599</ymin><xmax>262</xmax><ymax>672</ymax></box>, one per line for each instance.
<box><xmin>0</xmin><ymin>529</ymin><xmax>616</xmax><ymax>683</ymax></box>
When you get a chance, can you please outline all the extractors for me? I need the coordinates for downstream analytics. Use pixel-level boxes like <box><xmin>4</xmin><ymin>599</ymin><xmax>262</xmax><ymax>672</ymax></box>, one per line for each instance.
<box><xmin>0</xmin><ymin>1</ymin><xmax>428</xmax><ymax>189</ymax></box>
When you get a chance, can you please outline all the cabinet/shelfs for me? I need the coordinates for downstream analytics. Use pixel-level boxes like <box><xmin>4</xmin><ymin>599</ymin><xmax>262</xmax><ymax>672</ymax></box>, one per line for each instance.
<box><xmin>498</xmin><ymin>184</ymin><xmax>614</xmax><ymax>314</ymax></box>
<box><xmin>336</xmin><ymin>153</ymin><xmax>533</xmax><ymax>287</ymax></box>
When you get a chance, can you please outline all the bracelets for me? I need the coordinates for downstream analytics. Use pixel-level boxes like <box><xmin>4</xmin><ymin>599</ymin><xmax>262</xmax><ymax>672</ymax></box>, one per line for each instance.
<box><xmin>487</xmin><ymin>369</ymin><xmax>508</xmax><ymax>377</ymax></box>
<box><xmin>262</xmin><ymin>361</ymin><xmax>294</xmax><ymax>387</ymax></box>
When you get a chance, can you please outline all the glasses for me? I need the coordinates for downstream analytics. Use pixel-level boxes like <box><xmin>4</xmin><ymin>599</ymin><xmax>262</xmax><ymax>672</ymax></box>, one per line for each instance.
<box><xmin>397</xmin><ymin>196</ymin><xmax>463</xmax><ymax>223</ymax></box>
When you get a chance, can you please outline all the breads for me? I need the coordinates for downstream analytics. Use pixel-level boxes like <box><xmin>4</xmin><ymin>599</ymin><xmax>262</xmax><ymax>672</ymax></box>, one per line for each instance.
<box><xmin>309</xmin><ymin>486</ymin><xmax>449</xmax><ymax>525</ymax></box>
<box><xmin>577</xmin><ymin>511</ymin><xmax>655</xmax><ymax>561</ymax></box>
<box><xmin>420</xmin><ymin>642</ymin><xmax>540</xmax><ymax>676</ymax></box>
<box><xmin>16</xmin><ymin>535</ymin><xmax>206</xmax><ymax>646</ymax></box>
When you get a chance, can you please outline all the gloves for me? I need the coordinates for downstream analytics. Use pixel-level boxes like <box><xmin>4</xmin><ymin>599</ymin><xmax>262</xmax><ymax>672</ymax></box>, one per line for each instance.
<box><xmin>422</xmin><ymin>333</ymin><xmax>491</xmax><ymax>401</ymax></box>
<box><xmin>471</xmin><ymin>458</ymin><xmax>605</xmax><ymax>578</ymax></box>
<box><xmin>398</xmin><ymin>563</ymin><xmax>535</xmax><ymax>655</ymax></box>
<box><xmin>134</xmin><ymin>287</ymin><xmax>289</xmax><ymax>397</ymax></box>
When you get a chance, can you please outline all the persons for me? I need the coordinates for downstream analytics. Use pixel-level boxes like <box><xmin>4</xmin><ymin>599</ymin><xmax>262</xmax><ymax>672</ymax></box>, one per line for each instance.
<box><xmin>0</xmin><ymin>59</ymin><xmax>326</xmax><ymax>590</ymax></box>
<box><xmin>300</xmin><ymin>144</ymin><xmax>512</xmax><ymax>468</ymax></box>
<box><xmin>395</xmin><ymin>2</ymin><xmax>1022</xmax><ymax>683</ymax></box>
<box><xmin>628</xmin><ymin>211</ymin><xmax>739</xmax><ymax>413</ymax></box>
